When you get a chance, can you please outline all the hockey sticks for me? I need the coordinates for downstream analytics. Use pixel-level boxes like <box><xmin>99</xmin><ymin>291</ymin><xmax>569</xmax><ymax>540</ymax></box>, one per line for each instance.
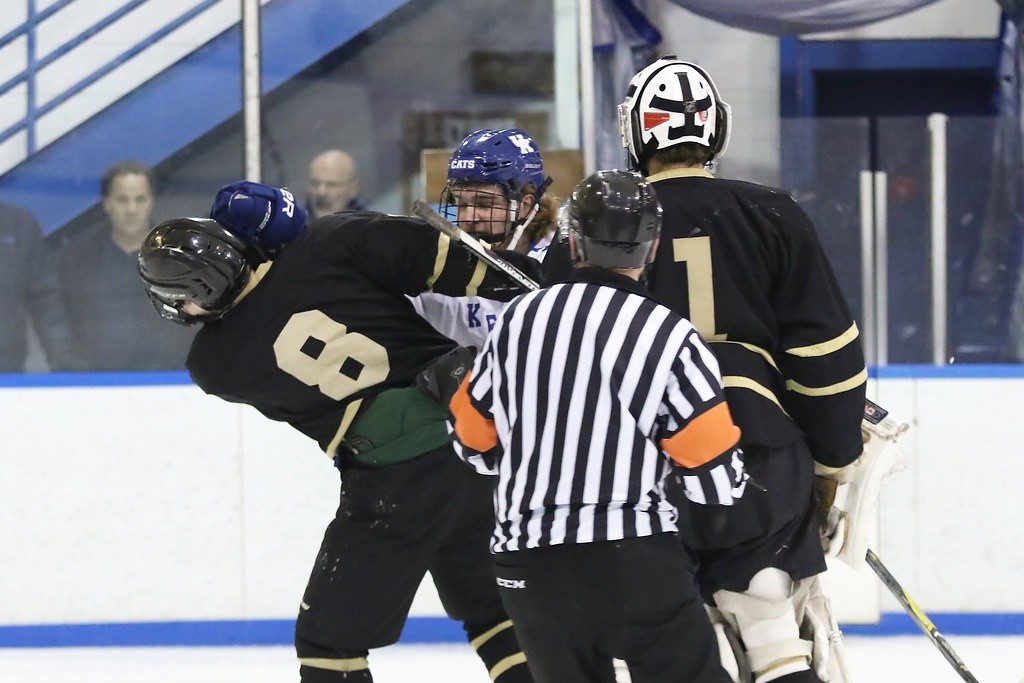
<box><xmin>862</xmin><ymin>547</ymin><xmax>979</xmax><ymax>683</ymax></box>
<box><xmin>410</xmin><ymin>199</ymin><xmax>543</xmax><ymax>292</ymax></box>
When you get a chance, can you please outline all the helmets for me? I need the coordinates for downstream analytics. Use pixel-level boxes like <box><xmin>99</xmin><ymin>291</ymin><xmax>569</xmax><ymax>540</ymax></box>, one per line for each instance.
<box><xmin>136</xmin><ymin>219</ymin><xmax>253</xmax><ymax>327</ymax></box>
<box><xmin>567</xmin><ymin>169</ymin><xmax>664</xmax><ymax>268</ymax></box>
<box><xmin>438</xmin><ymin>126</ymin><xmax>546</xmax><ymax>245</ymax></box>
<box><xmin>617</xmin><ymin>55</ymin><xmax>731</xmax><ymax>170</ymax></box>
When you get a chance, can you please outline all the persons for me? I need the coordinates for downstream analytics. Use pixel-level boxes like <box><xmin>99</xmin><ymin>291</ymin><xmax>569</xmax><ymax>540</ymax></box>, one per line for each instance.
<box><xmin>446</xmin><ymin>170</ymin><xmax>748</xmax><ymax>683</ymax></box>
<box><xmin>0</xmin><ymin>202</ymin><xmax>89</xmax><ymax>372</ymax></box>
<box><xmin>305</xmin><ymin>150</ymin><xmax>363</xmax><ymax>221</ymax></box>
<box><xmin>138</xmin><ymin>56</ymin><xmax>909</xmax><ymax>683</ymax></box>
<box><xmin>61</xmin><ymin>162</ymin><xmax>205</xmax><ymax>373</ymax></box>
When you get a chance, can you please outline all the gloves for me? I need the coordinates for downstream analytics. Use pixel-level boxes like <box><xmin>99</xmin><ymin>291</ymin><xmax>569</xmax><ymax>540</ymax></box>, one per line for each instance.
<box><xmin>211</xmin><ymin>180</ymin><xmax>307</xmax><ymax>254</ymax></box>
